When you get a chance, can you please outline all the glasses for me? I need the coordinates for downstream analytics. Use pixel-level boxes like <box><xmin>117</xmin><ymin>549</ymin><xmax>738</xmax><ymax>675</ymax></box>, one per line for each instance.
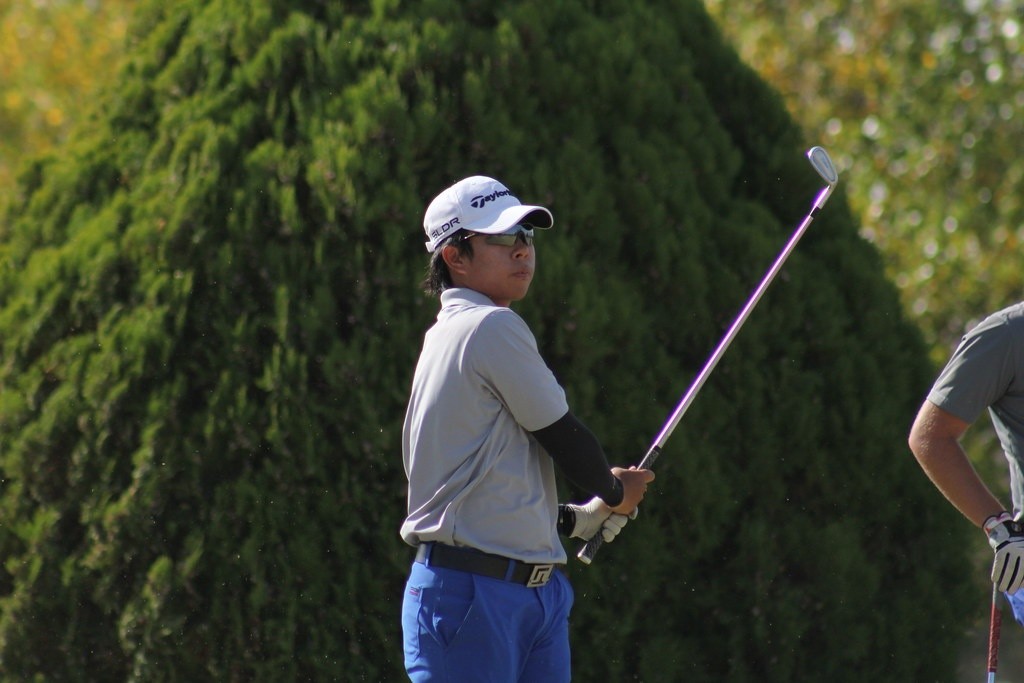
<box><xmin>459</xmin><ymin>223</ymin><xmax>535</xmax><ymax>247</ymax></box>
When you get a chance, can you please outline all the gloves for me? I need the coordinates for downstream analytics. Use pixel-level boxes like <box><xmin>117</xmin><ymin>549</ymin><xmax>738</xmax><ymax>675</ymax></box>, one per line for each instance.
<box><xmin>983</xmin><ymin>512</ymin><xmax>1024</xmax><ymax>595</ymax></box>
<box><xmin>565</xmin><ymin>495</ymin><xmax>639</xmax><ymax>541</ymax></box>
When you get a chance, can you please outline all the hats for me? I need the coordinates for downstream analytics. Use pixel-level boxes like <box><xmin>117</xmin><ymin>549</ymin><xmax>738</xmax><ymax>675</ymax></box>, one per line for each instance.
<box><xmin>423</xmin><ymin>175</ymin><xmax>554</xmax><ymax>253</ymax></box>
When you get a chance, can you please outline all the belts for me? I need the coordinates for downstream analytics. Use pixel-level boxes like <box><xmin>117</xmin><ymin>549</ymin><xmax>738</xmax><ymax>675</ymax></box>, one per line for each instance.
<box><xmin>414</xmin><ymin>541</ymin><xmax>555</xmax><ymax>588</ymax></box>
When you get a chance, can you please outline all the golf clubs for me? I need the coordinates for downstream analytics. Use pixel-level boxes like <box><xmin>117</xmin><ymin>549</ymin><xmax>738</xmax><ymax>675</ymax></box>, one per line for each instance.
<box><xmin>576</xmin><ymin>144</ymin><xmax>838</xmax><ymax>565</ymax></box>
<box><xmin>986</xmin><ymin>582</ymin><xmax>1000</xmax><ymax>683</ymax></box>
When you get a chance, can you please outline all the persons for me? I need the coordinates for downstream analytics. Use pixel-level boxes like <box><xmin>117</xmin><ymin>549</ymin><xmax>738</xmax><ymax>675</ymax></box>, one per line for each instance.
<box><xmin>906</xmin><ymin>301</ymin><xmax>1024</xmax><ymax>623</ymax></box>
<box><xmin>398</xmin><ymin>176</ymin><xmax>656</xmax><ymax>683</ymax></box>
